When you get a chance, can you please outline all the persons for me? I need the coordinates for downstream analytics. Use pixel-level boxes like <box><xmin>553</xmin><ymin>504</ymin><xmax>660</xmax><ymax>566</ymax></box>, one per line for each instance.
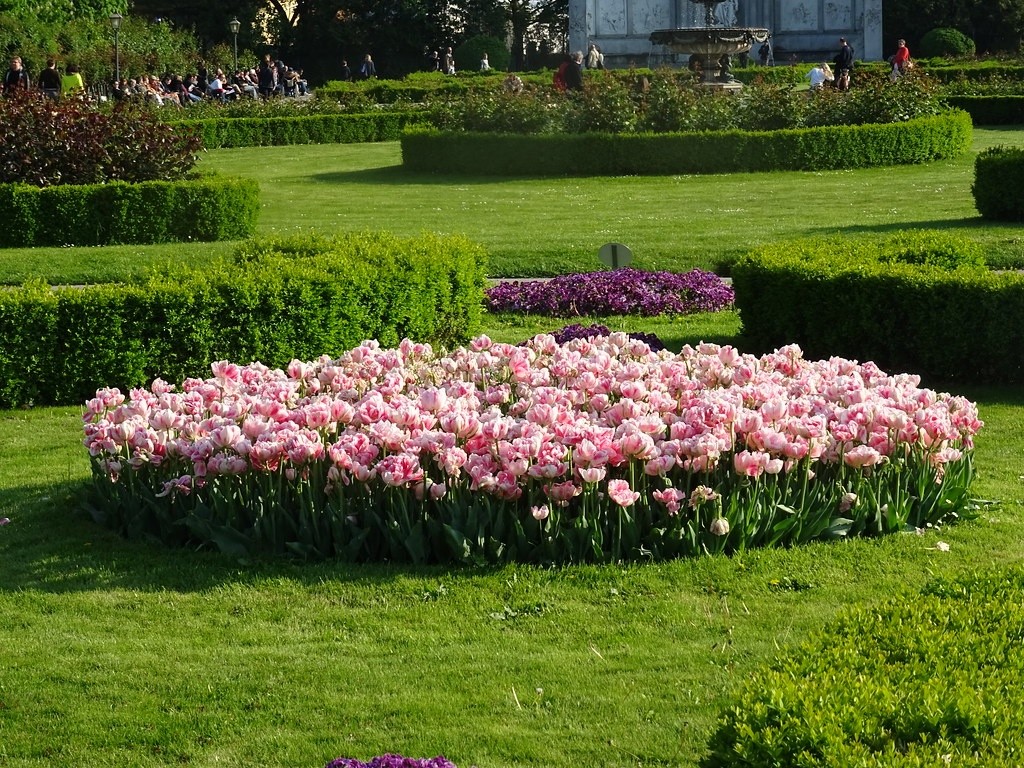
<box><xmin>61</xmin><ymin>64</ymin><xmax>84</xmax><ymax>99</ymax></box>
<box><xmin>428</xmin><ymin>51</ymin><xmax>442</xmax><ymax>72</ymax></box>
<box><xmin>444</xmin><ymin>47</ymin><xmax>453</xmax><ymax>74</ymax></box>
<box><xmin>360</xmin><ymin>54</ymin><xmax>376</xmax><ymax>78</ymax></box>
<box><xmin>3</xmin><ymin>55</ymin><xmax>30</xmax><ymax>94</ymax></box>
<box><xmin>553</xmin><ymin>52</ymin><xmax>575</xmax><ymax>95</ymax></box>
<box><xmin>112</xmin><ymin>54</ymin><xmax>311</xmax><ymax>109</ymax></box>
<box><xmin>448</xmin><ymin>61</ymin><xmax>455</xmax><ymax>75</ymax></box>
<box><xmin>339</xmin><ymin>61</ymin><xmax>352</xmax><ymax>82</ymax></box>
<box><xmin>38</xmin><ymin>58</ymin><xmax>62</xmax><ymax>100</ymax></box>
<box><xmin>833</xmin><ymin>38</ymin><xmax>853</xmax><ymax>88</ymax></box>
<box><xmin>596</xmin><ymin>47</ymin><xmax>605</xmax><ymax>70</ymax></box>
<box><xmin>565</xmin><ymin>50</ymin><xmax>584</xmax><ymax>91</ymax></box>
<box><xmin>480</xmin><ymin>53</ymin><xmax>491</xmax><ymax>71</ymax></box>
<box><xmin>805</xmin><ymin>62</ymin><xmax>850</xmax><ymax>93</ymax></box>
<box><xmin>890</xmin><ymin>39</ymin><xmax>910</xmax><ymax>77</ymax></box>
<box><xmin>588</xmin><ymin>45</ymin><xmax>599</xmax><ymax>69</ymax></box>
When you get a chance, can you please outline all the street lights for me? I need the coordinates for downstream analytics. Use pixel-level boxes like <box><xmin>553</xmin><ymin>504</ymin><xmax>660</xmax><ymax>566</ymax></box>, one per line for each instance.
<box><xmin>108</xmin><ymin>8</ymin><xmax>123</xmax><ymax>82</ymax></box>
<box><xmin>229</xmin><ymin>15</ymin><xmax>242</xmax><ymax>74</ymax></box>
